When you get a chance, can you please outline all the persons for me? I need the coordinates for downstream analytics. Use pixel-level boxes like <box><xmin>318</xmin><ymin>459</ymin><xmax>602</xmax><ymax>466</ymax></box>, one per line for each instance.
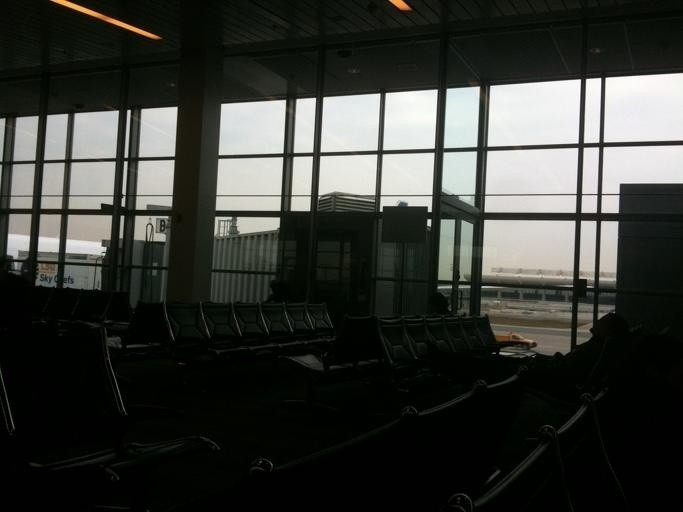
<box><xmin>428</xmin><ymin>293</ymin><xmax>450</xmax><ymax>315</ymax></box>
<box><xmin>265</xmin><ymin>281</ymin><xmax>292</xmax><ymax>303</ymax></box>
<box><xmin>502</xmin><ymin>314</ymin><xmax>629</xmax><ymax>402</ymax></box>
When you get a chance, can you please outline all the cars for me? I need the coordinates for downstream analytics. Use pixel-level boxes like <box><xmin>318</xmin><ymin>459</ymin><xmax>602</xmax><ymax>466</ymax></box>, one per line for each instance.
<box><xmin>493</xmin><ymin>332</ymin><xmax>537</xmax><ymax>352</ymax></box>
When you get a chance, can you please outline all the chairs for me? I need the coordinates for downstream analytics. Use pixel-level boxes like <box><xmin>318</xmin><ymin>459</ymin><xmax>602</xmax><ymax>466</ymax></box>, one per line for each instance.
<box><xmin>0</xmin><ymin>283</ymin><xmax>672</xmax><ymax>510</ymax></box>
